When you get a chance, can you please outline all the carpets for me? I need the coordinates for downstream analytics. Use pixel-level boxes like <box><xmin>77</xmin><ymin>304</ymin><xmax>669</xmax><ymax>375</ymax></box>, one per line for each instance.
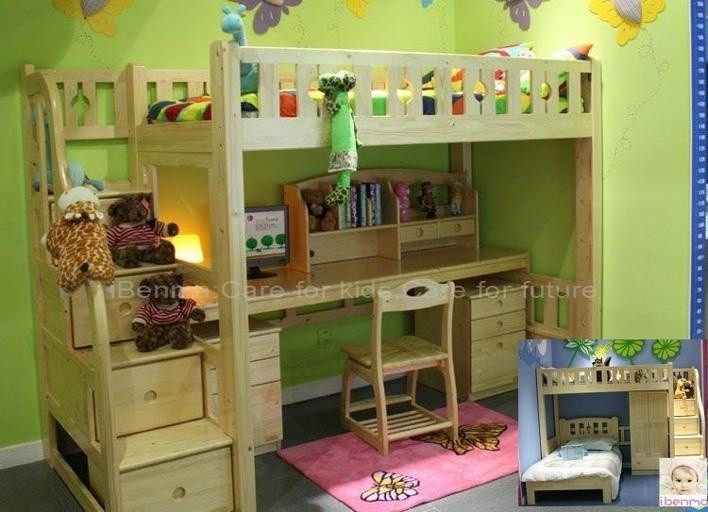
<box><xmin>276</xmin><ymin>400</ymin><xmax>519</xmax><ymax>512</ymax></box>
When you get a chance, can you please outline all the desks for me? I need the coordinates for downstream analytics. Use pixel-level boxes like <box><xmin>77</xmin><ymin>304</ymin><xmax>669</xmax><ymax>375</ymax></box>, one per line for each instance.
<box><xmin>170</xmin><ymin>165</ymin><xmax>537</xmax><ymax>464</ymax></box>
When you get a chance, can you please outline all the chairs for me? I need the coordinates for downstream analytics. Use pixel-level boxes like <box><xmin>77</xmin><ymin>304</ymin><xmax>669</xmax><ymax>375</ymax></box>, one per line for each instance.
<box><xmin>340</xmin><ymin>278</ymin><xmax>460</xmax><ymax>457</ymax></box>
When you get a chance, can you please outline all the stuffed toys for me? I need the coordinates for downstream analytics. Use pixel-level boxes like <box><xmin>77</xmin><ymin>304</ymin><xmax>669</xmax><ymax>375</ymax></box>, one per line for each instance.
<box><xmin>592</xmin><ymin>356</ymin><xmax>612</xmax><ymax>382</ymax></box>
<box><xmin>675</xmin><ymin>378</ymin><xmax>689</xmax><ymax>400</ymax></box>
<box><xmin>220</xmin><ymin>4</ymin><xmax>258</xmax><ymax>94</ymax></box>
<box><xmin>31</xmin><ymin>163</ymin><xmax>104</xmax><ymax>193</ymax></box>
<box><xmin>685</xmin><ymin>380</ymin><xmax>695</xmax><ymax>399</ymax></box>
<box><xmin>39</xmin><ymin>187</ymin><xmax>114</xmax><ymax>300</ymax></box>
<box><xmin>395</xmin><ymin>182</ymin><xmax>410</xmax><ymax>222</ymax></box>
<box><xmin>300</xmin><ymin>188</ymin><xmax>337</xmax><ymax>231</ymax></box>
<box><xmin>106</xmin><ymin>193</ymin><xmax>179</xmax><ymax>268</ymax></box>
<box><xmin>131</xmin><ymin>270</ymin><xmax>206</xmax><ymax>352</ymax></box>
<box><xmin>317</xmin><ymin>70</ymin><xmax>362</xmax><ymax>206</ymax></box>
<box><xmin>450</xmin><ymin>181</ymin><xmax>464</xmax><ymax>215</ymax></box>
<box><xmin>417</xmin><ymin>181</ymin><xmax>437</xmax><ymax>218</ymax></box>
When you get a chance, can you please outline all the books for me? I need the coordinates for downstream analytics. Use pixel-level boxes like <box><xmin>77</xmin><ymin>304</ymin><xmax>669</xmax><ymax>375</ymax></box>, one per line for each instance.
<box><xmin>332</xmin><ymin>181</ymin><xmax>382</xmax><ymax>228</ymax></box>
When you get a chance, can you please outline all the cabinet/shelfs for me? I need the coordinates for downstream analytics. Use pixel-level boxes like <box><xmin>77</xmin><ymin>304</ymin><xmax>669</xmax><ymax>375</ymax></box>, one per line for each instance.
<box><xmin>629</xmin><ymin>391</ymin><xmax>669</xmax><ymax>477</ymax></box>
<box><xmin>413</xmin><ymin>278</ymin><xmax>528</xmax><ymax>403</ymax></box>
<box><xmin>191</xmin><ymin>315</ymin><xmax>284</xmax><ymax>458</ymax></box>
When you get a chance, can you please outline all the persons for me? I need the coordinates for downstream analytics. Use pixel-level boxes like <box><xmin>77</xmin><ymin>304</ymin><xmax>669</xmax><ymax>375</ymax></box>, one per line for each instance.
<box><xmin>662</xmin><ymin>458</ymin><xmax>707</xmax><ymax>496</ymax></box>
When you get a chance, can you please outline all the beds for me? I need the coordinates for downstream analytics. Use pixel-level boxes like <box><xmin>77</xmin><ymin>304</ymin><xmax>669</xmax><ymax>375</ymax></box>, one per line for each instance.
<box><xmin>19</xmin><ymin>38</ymin><xmax>604</xmax><ymax>512</ymax></box>
<box><xmin>535</xmin><ymin>361</ymin><xmax>705</xmax><ymax>477</ymax></box>
<box><xmin>521</xmin><ymin>416</ymin><xmax>623</xmax><ymax>506</ymax></box>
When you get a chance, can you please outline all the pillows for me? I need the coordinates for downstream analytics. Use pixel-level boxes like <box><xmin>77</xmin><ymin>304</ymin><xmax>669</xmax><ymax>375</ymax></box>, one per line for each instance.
<box><xmin>565</xmin><ymin>436</ymin><xmax>618</xmax><ymax>452</ymax></box>
<box><xmin>452</xmin><ymin>39</ymin><xmax>592</xmax><ymax>98</ymax></box>
